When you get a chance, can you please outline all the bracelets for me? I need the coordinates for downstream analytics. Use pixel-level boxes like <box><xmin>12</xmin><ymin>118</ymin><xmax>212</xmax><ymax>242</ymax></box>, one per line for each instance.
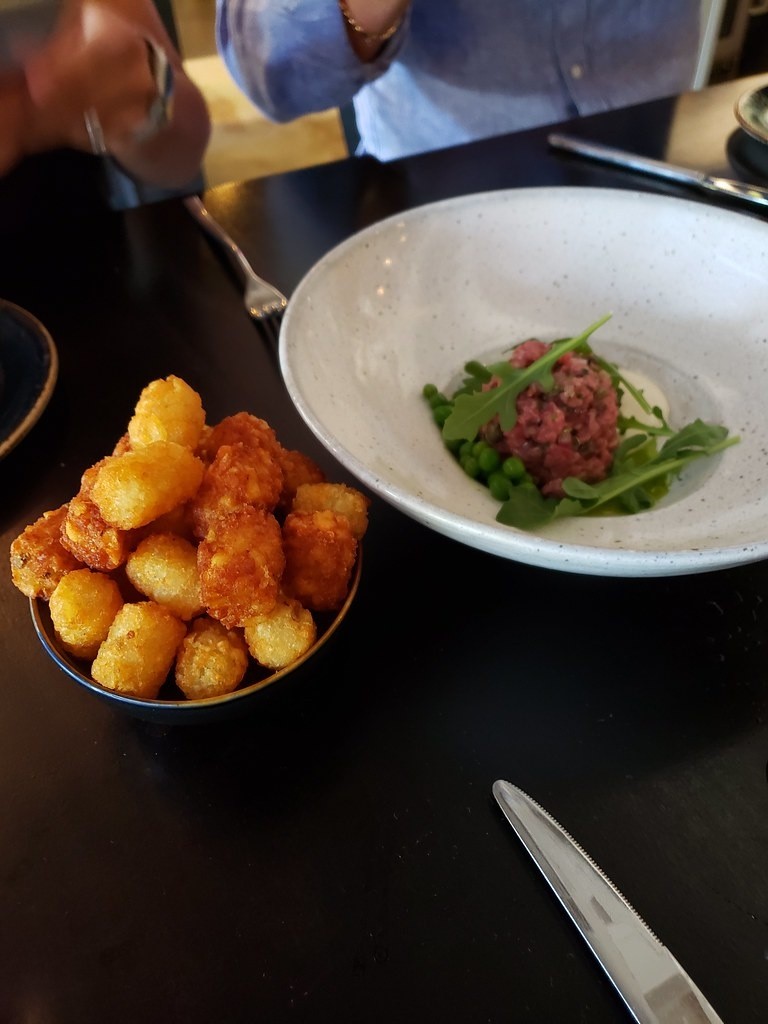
<box><xmin>338</xmin><ymin>0</ymin><xmax>402</xmax><ymax>48</ymax></box>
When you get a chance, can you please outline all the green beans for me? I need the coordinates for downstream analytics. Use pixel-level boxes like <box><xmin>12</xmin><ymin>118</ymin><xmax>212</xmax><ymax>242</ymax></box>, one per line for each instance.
<box><xmin>423</xmin><ymin>384</ymin><xmax>538</xmax><ymax>503</ymax></box>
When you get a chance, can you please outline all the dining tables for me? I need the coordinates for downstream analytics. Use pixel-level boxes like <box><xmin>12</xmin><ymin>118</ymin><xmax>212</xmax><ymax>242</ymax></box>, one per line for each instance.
<box><xmin>0</xmin><ymin>71</ymin><xmax>768</xmax><ymax>1024</ymax></box>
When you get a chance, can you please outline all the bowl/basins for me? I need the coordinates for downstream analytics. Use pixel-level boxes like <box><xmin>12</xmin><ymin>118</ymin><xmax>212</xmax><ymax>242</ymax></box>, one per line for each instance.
<box><xmin>31</xmin><ymin>478</ymin><xmax>364</xmax><ymax>709</ymax></box>
<box><xmin>277</xmin><ymin>184</ymin><xmax>768</xmax><ymax>576</ymax></box>
<box><xmin>0</xmin><ymin>299</ymin><xmax>62</xmax><ymax>464</ymax></box>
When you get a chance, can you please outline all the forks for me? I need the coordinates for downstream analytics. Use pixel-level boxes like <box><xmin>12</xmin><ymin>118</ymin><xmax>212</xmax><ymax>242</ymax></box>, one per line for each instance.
<box><xmin>183</xmin><ymin>192</ymin><xmax>289</xmax><ymax>320</ymax></box>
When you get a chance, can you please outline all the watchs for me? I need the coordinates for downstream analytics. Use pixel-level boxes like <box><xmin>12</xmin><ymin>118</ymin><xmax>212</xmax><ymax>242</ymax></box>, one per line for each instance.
<box><xmin>84</xmin><ymin>33</ymin><xmax>173</xmax><ymax>155</ymax></box>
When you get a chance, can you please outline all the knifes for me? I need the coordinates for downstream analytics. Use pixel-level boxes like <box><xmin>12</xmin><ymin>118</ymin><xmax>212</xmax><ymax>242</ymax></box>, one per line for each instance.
<box><xmin>491</xmin><ymin>780</ymin><xmax>724</xmax><ymax>1024</ymax></box>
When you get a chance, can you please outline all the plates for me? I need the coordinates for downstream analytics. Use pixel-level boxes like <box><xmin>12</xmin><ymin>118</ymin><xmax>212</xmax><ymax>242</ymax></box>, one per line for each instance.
<box><xmin>733</xmin><ymin>82</ymin><xmax>768</xmax><ymax>146</ymax></box>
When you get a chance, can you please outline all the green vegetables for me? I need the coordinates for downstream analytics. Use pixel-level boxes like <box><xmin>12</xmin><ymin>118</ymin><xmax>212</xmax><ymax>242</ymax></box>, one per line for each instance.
<box><xmin>440</xmin><ymin>309</ymin><xmax>741</xmax><ymax>531</ymax></box>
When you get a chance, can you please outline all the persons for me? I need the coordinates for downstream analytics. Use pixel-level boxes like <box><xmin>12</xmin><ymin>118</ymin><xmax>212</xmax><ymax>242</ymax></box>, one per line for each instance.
<box><xmin>0</xmin><ymin>0</ymin><xmax>213</xmax><ymax>235</ymax></box>
<box><xmin>215</xmin><ymin>0</ymin><xmax>705</xmax><ymax>161</ymax></box>
